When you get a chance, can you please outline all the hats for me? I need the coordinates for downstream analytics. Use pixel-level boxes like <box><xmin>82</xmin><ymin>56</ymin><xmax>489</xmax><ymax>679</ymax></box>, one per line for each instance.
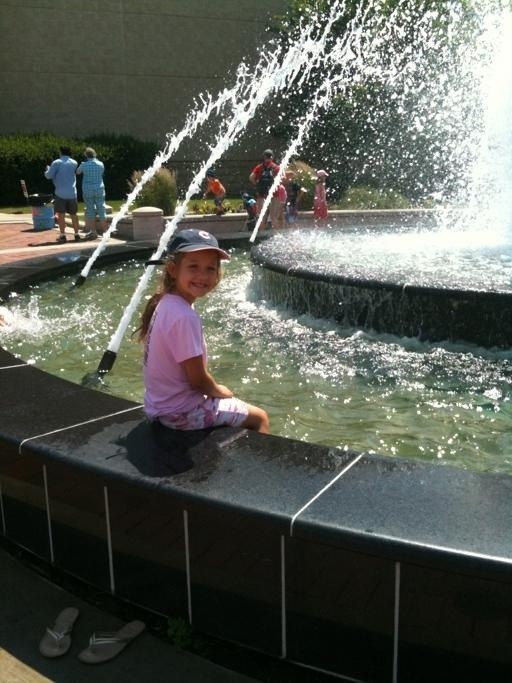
<box><xmin>263</xmin><ymin>149</ymin><xmax>273</xmax><ymax>156</ymax></box>
<box><xmin>317</xmin><ymin>170</ymin><xmax>329</xmax><ymax>177</ymax></box>
<box><xmin>167</xmin><ymin>228</ymin><xmax>231</xmax><ymax>260</ymax></box>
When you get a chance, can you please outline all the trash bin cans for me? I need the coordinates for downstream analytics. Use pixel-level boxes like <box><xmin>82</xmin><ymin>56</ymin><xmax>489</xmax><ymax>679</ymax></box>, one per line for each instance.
<box><xmin>28</xmin><ymin>193</ymin><xmax>55</xmax><ymax>231</ymax></box>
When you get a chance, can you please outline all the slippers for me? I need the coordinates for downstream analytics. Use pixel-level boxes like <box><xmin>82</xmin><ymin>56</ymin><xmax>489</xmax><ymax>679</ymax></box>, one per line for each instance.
<box><xmin>77</xmin><ymin>620</ymin><xmax>146</xmax><ymax>665</ymax></box>
<box><xmin>40</xmin><ymin>607</ymin><xmax>79</xmax><ymax>657</ymax></box>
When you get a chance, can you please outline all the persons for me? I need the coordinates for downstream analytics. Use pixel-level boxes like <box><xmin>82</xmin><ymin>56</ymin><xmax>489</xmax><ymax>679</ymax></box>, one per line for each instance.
<box><xmin>45</xmin><ymin>145</ymin><xmax>80</xmax><ymax>241</ymax></box>
<box><xmin>76</xmin><ymin>147</ymin><xmax>108</xmax><ymax>239</ymax></box>
<box><xmin>130</xmin><ymin>228</ymin><xmax>270</xmax><ymax>434</ymax></box>
<box><xmin>203</xmin><ymin>148</ymin><xmax>330</xmax><ymax>232</ymax></box>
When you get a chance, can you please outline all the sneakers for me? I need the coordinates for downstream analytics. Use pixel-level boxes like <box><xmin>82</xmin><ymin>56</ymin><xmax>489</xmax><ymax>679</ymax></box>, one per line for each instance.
<box><xmin>56</xmin><ymin>233</ymin><xmax>97</xmax><ymax>242</ymax></box>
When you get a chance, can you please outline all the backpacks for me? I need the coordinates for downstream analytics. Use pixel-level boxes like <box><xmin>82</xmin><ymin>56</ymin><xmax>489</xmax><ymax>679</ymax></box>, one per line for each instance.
<box><xmin>256</xmin><ymin>164</ymin><xmax>275</xmax><ymax>197</ymax></box>
